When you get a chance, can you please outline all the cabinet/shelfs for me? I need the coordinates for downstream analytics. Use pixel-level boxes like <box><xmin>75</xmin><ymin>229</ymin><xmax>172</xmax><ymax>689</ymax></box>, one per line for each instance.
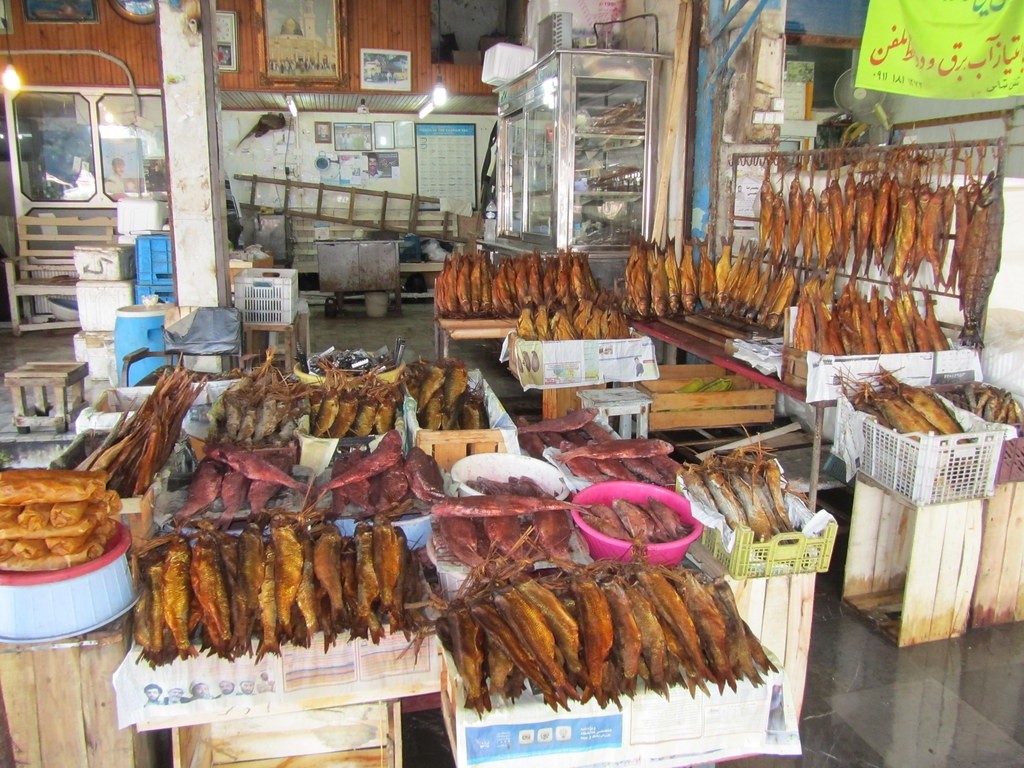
<box><xmin>314</xmin><ymin>239</ymin><xmax>402</xmax><ymax>315</ymax></box>
<box><xmin>476</xmin><ymin>48</ymin><xmax>673</xmax><ymax>293</ymax></box>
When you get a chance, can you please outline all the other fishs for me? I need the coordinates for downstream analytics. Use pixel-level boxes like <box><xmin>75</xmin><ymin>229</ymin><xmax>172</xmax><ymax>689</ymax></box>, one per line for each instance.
<box><xmin>72</xmin><ymin>351</ymin><xmax>208</xmax><ymax>499</ymax></box>
<box><xmin>208</xmin><ymin>358</ymin><xmax>492</xmax><ymax>446</ymax></box>
<box><xmin>620</xmin><ymin>228</ymin><xmax>757</xmax><ymax>325</ymax></box>
<box><xmin>170</xmin><ymin>429</ymin><xmax>449</xmax><ymax>527</ymax></box>
<box><xmin>847</xmin><ymin>373</ymin><xmax>1024</xmax><ymax>446</ymax></box>
<box><xmin>430</xmin><ymin>476</ymin><xmax>605</xmax><ymax>565</ymax></box>
<box><xmin>514</xmin><ymin>407</ymin><xmax>794</xmax><ymax>549</ymax></box>
<box><xmin>756</xmin><ymin>172</ymin><xmax>1004</xmax><ymax>355</ymax></box>
<box><xmin>433</xmin><ymin>560</ymin><xmax>778</xmax><ymax>720</ymax></box>
<box><xmin>126</xmin><ymin>516</ymin><xmax>429</xmax><ymax>667</ymax></box>
<box><xmin>435</xmin><ymin>246</ymin><xmax>632</xmax><ymax>341</ymax></box>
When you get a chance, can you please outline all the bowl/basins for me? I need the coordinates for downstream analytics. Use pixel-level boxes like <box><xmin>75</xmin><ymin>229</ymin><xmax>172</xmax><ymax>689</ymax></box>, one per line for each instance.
<box><xmin>570</xmin><ymin>478</ymin><xmax>704</xmax><ymax>570</ymax></box>
<box><xmin>449</xmin><ymin>451</ymin><xmax>570</xmax><ymax>501</ymax></box>
<box><xmin>47</xmin><ymin>298</ymin><xmax>78</xmax><ymax>321</ymax></box>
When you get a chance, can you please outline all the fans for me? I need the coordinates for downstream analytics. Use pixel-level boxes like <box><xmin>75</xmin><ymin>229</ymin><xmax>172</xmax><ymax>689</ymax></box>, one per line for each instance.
<box><xmin>832</xmin><ymin>66</ymin><xmax>887</xmax><ymax>145</ymax></box>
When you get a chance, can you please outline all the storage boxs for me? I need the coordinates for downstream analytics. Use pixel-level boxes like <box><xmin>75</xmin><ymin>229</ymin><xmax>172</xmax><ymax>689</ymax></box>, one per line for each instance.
<box><xmin>70</xmin><ymin>232</ymin><xmax>177</xmax><ymax>333</ymax></box>
<box><xmin>638</xmin><ymin>363</ymin><xmax>777</xmax><ymax>431</ymax></box>
<box><xmin>828</xmin><ymin>395</ymin><xmax>1023</xmax><ymax>647</ymax></box>
<box><xmin>229</xmin><ymin>250</ymin><xmax>298</xmax><ymax>325</ymax></box>
<box><xmin>73</xmin><ymin>331</ymin><xmax>113</xmax><ymax>379</ymax></box>
<box><xmin>76</xmin><ymin>369</ymin><xmax>520</xmax><ymax>473</ymax></box>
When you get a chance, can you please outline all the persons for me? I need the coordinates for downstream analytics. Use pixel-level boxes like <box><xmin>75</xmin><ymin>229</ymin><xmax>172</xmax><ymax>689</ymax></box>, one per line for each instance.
<box><xmin>634</xmin><ymin>357</ymin><xmax>644</xmax><ymax>377</ymax></box>
<box><xmin>318</xmin><ymin>126</ymin><xmax>329</xmax><ymax>139</ymax></box>
<box><xmin>367</xmin><ymin>153</ymin><xmax>383</xmax><ymax>178</ymax></box>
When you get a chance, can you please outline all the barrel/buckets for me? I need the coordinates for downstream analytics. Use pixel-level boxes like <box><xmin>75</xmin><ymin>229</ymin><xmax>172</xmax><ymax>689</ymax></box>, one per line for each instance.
<box><xmin>365</xmin><ymin>291</ymin><xmax>389</xmax><ymax>318</ymax></box>
<box><xmin>114</xmin><ymin>303</ymin><xmax>179</xmax><ymax>387</ymax></box>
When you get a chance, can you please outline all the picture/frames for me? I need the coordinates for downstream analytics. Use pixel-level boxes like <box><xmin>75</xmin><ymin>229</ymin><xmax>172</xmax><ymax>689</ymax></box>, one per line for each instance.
<box><xmin>333</xmin><ymin>123</ymin><xmax>372</xmax><ymax>151</ymax></box>
<box><xmin>21</xmin><ymin>0</ymin><xmax>101</xmax><ymax>25</ymax></box>
<box><xmin>362</xmin><ymin>151</ymin><xmax>399</xmax><ymax>179</ymax></box>
<box><xmin>0</xmin><ymin>0</ymin><xmax>14</xmax><ymax>35</ymax></box>
<box><xmin>107</xmin><ymin>0</ymin><xmax>155</xmax><ymax>24</ymax></box>
<box><xmin>315</xmin><ymin>122</ymin><xmax>332</xmax><ymax>143</ymax></box>
<box><xmin>249</xmin><ymin>0</ymin><xmax>350</xmax><ymax>91</ymax></box>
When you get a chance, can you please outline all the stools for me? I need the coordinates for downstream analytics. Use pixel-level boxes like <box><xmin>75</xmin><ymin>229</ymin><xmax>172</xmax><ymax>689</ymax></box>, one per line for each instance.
<box><xmin>577</xmin><ymin>387</ymin><xmax>654</xmax><ymax>443</ymax></box>
<box><xmin>240</xmin><ymin>317</ymin><xmax>300</xmax><ymax>370</ymax></box>
<box><xmin>5</xmin><ymin>361</ymin><xmax>88</xmax><ymax>433</ymax></box>
